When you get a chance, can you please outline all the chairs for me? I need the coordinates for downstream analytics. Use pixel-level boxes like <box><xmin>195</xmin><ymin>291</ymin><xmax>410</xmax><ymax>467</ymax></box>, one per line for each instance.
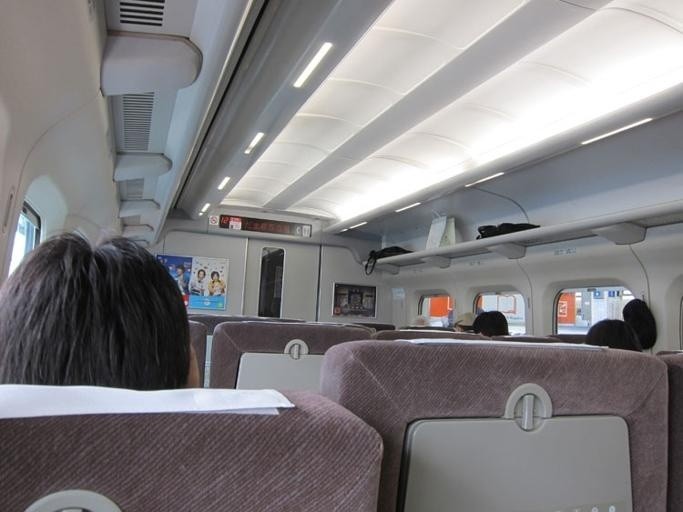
<box><xmin>0</xmin><ymin>312</ymin><xmax>683</xmax><ymax>512</ymax></box>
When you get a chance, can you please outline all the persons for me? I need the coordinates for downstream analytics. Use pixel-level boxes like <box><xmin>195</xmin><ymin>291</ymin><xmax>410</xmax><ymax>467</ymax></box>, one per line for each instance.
<box><xmin>473</xmin><ymin>309</ymin><xmax>511</xmax><ymax>336</ymax></box>
<box><xmin>584</xmin><ymin>318</ymin><xmax>644</xmax><ymax>353</ymax></box>
<box><xmin>453</xmin><ymin>312</ymin><xmax>479</xmax><ymax>335</ymax></box>
<box><xmin>191</xmin><ymin>268</ymin><xmax>207</xmax><ymax>296</ymax></box>
<box><xmin>173</xmin><ymin>265</ymin><xmax>190</xmax><ymax>307</ymax></box>
<box><xmin>1</xmin><ymin>229</ymin><xmax>202</xmax><ymax>390</ymax></box>
<box><xmin>409</xmin><ymin>314</ymin><xmax>432</xmax><ymax>326</ymax></box>
<box><xmin>207</xmin><ymin>270</ymin><xmax>226</xmax><ymax>297</ymax></box>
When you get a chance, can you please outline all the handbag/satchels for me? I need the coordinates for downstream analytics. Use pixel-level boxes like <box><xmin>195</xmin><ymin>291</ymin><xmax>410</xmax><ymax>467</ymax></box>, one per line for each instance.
<box><xmin>364</xmin><ymin>245</ymin><xmax>413</xmax><ymax>277</ymax></box>
<box><xmin>475</xmin><ymin>221</ymin><xmax>540</xmax><ymax>242</ymax></box>
